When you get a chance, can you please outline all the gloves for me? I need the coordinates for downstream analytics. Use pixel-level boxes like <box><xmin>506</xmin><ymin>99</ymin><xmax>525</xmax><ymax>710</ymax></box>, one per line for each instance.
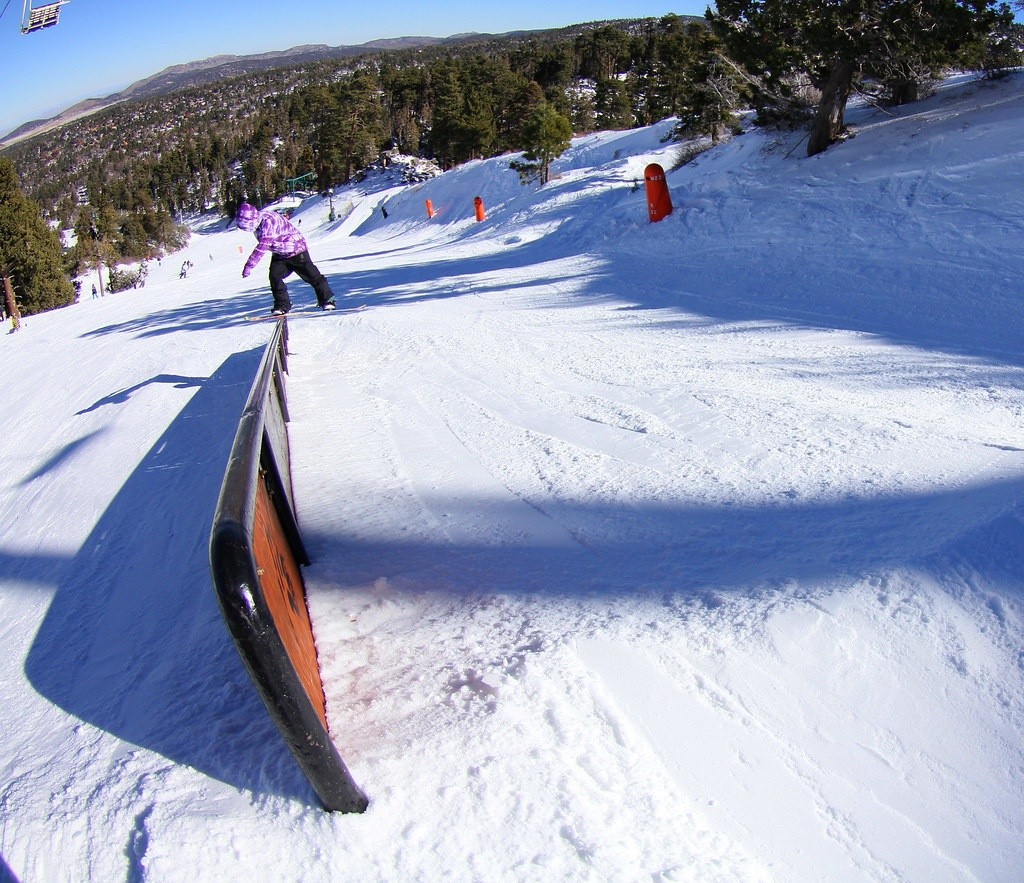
<box><xmin>242</xmin><ymin>264</ymin><xmax>251</xmax><ymax>278</ymax></box>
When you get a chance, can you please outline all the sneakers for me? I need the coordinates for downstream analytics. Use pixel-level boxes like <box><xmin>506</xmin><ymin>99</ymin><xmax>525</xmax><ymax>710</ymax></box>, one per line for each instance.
<box><xmin>271</xmin><ymin>308</ymin><xmax>289</xmax><ymax>316</ymax></box>
<box><xmin>323</xmin><ymin>300</ymin><xmax>336</xmax><ymax>310</ymax></box>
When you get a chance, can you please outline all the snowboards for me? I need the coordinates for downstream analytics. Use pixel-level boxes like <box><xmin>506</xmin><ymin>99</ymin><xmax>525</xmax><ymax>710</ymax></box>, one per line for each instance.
<box><xmin>245</xmin><ymin>303</ymin><xmax>367</xmax><ymax>322</ymax></box>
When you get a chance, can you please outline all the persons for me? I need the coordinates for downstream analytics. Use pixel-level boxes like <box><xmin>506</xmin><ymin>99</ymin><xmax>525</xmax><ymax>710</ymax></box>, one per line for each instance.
<box><xmin>133</xmin><ymin>256</ymin><xmax>161</xmax><ymax>289</ymax></box>
<box><xmin>180</xmin><ymin>261</ymin><xmax>191</xmax><ymax>278</ymax></box>
<box><xmin>92</xmin><ymin>284</ymin><xmax>98</xmax><ymax>299</ymax></box>
<box><xmin>236</xmin><ymin>203</ymin><xmax>335</xmax><ymax>316</ymax></box>
